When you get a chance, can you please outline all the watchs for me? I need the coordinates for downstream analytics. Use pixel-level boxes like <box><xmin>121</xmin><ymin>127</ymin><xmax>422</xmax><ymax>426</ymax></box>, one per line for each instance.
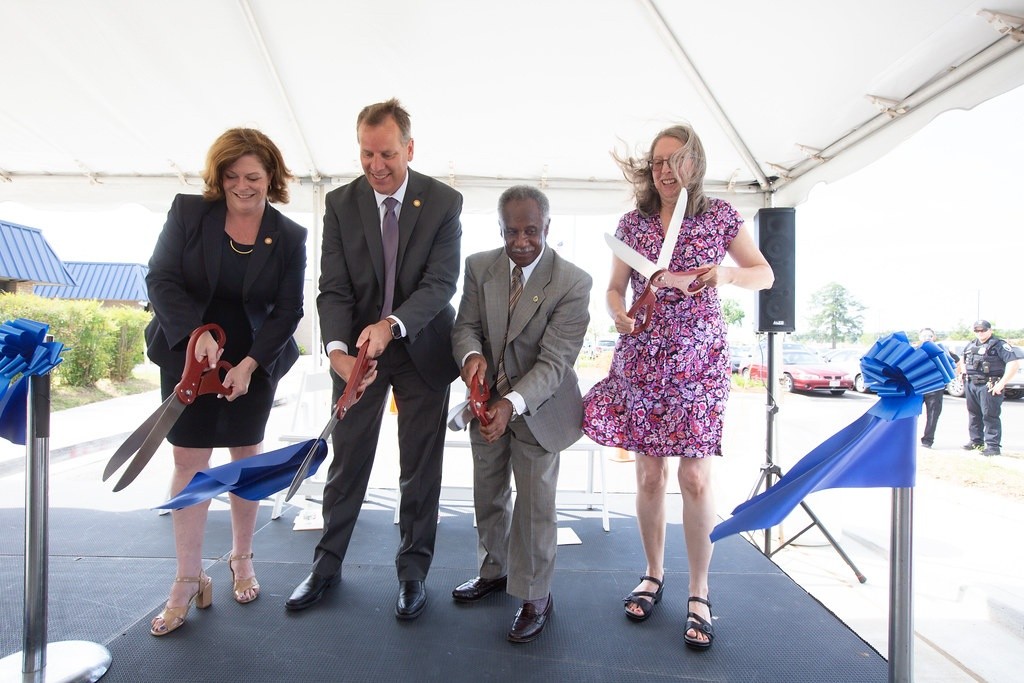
<box><xmin>385</xmin><ymin>317</ymin><xmax>402</xmax><ymax>339</ymax></box>
<box><xmin>510</xmin><ymin>404</ymin><xmax>517</xmax><ymax>422</ymax></box>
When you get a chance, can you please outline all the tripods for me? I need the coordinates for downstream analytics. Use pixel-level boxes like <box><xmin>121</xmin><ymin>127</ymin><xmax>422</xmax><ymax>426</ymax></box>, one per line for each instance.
<box><xmin>744</xmin><ymin>333</ymin><xmax>866</xmax><ymax>584</ymax></box>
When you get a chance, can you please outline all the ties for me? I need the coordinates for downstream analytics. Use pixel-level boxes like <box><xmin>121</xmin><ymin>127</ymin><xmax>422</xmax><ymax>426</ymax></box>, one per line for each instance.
<box><xmin>496</xmin><ymin>265</ymin><xmax>523</xmax><ymax>399</ymax></box>
<box><xmin>379</xmin><ymin>197</ymin><xmax>398</xmax><ymax>322</ymax></box>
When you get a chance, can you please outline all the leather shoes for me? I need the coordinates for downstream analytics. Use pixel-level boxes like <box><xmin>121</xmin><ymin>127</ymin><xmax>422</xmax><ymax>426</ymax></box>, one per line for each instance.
<box><xmin>394</xmin><ymin>581</ymin><xmax>428</xmax><ymax>618</ymax></box>
<box><xmin>452</xmin><ymin>574</ymin><xmax>507</xmax><ymax>603</ymax></box>
<box><xmin>506</xmin><ymin>593</ymin><xmax>553</xmax><ymax>642</ymax></box>
<box><xmin>284</xmin><ymin>572</ymin><xmax>332</xmax><ymax>611</ymax></box>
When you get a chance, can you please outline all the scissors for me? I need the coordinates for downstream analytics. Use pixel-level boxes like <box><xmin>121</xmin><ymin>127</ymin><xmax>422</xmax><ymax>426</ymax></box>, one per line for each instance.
<box><xmin>284</xmin><ymin>340</ymin><xmax>370</xmax><ymax>503</ymax></box>
<box><xmin>102</xmin><ymin>323</ymin><xmax>234</xmax><ymax>493</ymax></box>
<box><xmin>603</xmin><ymin>188</ymin><xmax>710</xmax><ymax>335</ymax></box>
<box><xmin>447</xmin><ymin>374</ymin><xmax>491</xmax><ymax>432</ymax></box>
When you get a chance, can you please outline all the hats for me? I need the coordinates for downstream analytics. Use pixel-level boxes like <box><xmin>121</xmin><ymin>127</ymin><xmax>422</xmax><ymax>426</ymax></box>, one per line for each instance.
<box><xmin>973</xmin><ymin>320</ymin><xmax>990</xmax><ymax>328</ymax></box>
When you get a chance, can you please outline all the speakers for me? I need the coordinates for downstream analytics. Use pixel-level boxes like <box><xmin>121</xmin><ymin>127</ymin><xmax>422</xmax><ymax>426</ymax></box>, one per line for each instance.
<box><xmin>753</xmin><ymin>207</ymin><xmax>795</xmax><ymax>335</ymax></box>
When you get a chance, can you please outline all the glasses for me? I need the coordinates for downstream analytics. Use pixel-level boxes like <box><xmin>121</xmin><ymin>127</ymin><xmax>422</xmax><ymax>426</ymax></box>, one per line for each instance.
<box><xmin>974</xmin><ymin>329</ymin><xmax>988</xmax><ymax>332</ymax></box>
<box><xmin>649</xmin><ymin>157</ymin><xmax>697</xmax><ymax>172</ymax></box>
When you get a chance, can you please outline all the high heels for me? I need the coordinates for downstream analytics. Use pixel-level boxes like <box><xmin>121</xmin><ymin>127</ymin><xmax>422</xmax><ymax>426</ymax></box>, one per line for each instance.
<box><xmin>149</xmin><ymin>569</ymin><xmax>212</xmax><ymax>636</ymax></box>
<box><xmin>684</xmin><ymin>595</ymin><xmax>714</xmax><ymax>651</ymax></box>
<box><xmin>228</xmin><ymin>552</ymin><xmax>260</xmax><ymax>604</ymax></box>
<box><xmin>622</xmin><ymin>574</ymin><xmax>664</xmax><ymax>622</ymax></box>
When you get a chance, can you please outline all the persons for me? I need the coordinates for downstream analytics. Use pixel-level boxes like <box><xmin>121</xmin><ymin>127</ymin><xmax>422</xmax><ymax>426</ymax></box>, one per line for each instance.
<box><xmin>961</xmin><ymin>320</ymin><xmax>1018</xmax><ymax>456</ymax></box>
<box><xmin>142</xmin><ymin>129</ymin><xmax>307</xmax><ymax>634</ymax></box>
<box><xmin>283</xmin><ymin>100</ymin><xmax>462</xmax><ymax>619</ymax></box>
<box><xmin>451</xmin><ymin>185</ymin><xmax>593</xmax><ymax>644</ymax></box>
<box><xmin>913</xmin><ymin>327</ymin><xmax>960</xmax><ymax>449</ymax></box>
<box><xmin>578</xmin><ymin>123</ymin><xmax>775</xmax><ymax>649</ymax></box>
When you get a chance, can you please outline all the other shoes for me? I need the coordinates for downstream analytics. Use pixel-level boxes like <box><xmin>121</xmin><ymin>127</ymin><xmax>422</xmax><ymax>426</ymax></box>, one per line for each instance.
<box><xmin>922</xmin><ymin>441</ymin><xmax>931</xmax><ymax>447</ymax></box>
<box><xmin>963</xmin><ymin>442</ymin><xmax>984</xmax><ymax>449</ymax></box>
<box><xmin>981</xmin><ymin>448</ymin><xmax>1001</xmax><ymax>455</ymax></box>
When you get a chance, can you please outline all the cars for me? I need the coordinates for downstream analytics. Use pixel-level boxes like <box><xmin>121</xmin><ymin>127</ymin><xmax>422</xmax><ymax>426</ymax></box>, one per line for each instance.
<box><xmin>587</xmin><ymin>339</ymin><xmax>618</xmax><ymax>362</ymax></box>
<box><xmin>737</xmin><ymin>347</ymin><xmax>854</xmax><ymax>396</ymax></box>
<box><xmin>816</xmin><ymin>346</ymin><xmax>1024</xmax><ymax>399</ymax></box>
<box><xmin>727</xmin><ymin>348</ymin><xmax>750</xmax><ymax>374</ymax></box>
